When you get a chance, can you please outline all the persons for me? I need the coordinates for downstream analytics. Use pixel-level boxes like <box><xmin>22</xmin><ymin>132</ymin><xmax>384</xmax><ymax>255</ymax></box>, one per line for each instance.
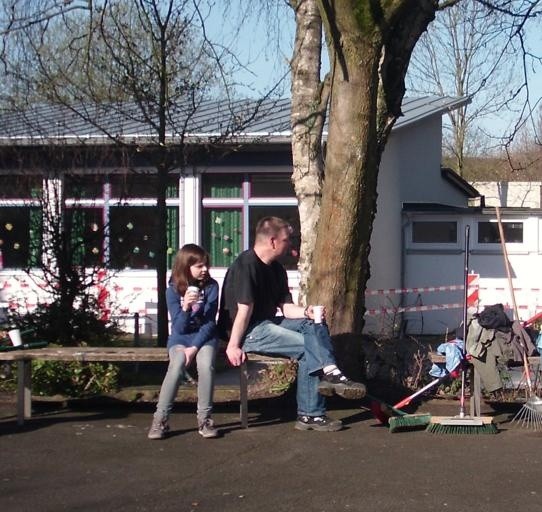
<box><xmin>217</xmin><ymin>216</ymin><xmax>366</xmax><ymax>432</ymax></box>
<box><xmin>148</xmin><ymin>244</ymin><xmax>219</xmax><ymax>439</ymax></box>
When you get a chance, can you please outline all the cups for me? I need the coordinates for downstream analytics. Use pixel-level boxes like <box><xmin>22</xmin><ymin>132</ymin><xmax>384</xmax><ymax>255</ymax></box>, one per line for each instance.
<box><xmin>313</xmin><ymin>306</ymin><xmax>325</xmax><ymax>324</ymax></box>
<box><xmin>187</xmin><ymin>286</ymin><xmax>200</xmax><ymax>301</ymax></box>
<box><xmin>7</xmin><ymin>329</ymin><xmax>23</xmax><ymax>348</ymax></box>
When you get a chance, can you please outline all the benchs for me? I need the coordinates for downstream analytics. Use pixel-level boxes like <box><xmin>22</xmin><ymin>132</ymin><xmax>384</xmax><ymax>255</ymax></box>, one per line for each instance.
<box><xmin>0</xmin><ymin>345</ymin><xmax>250</xmax><ymax>429</ymax></box>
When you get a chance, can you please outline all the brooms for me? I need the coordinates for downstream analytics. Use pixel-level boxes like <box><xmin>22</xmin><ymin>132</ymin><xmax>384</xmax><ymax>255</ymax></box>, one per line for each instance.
<box><xmin>365</xmin><ymin>394</ymin><xmax>431</xmax><ymax>434</ymax></box>
<box><xmin>424</xmin><ymin>224</ymin><xmax>499</xmax><ymax>435</ymax></box>
<box><xmin>370</xmin><ymin>354</ymin><xmax>472</xmax><ymax>425</ymax></box>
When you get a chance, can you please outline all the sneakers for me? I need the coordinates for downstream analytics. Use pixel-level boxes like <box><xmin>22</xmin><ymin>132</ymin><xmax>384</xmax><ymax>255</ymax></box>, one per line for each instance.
<box><xmin>148</xmin><ymin>420</ymin><xmax>170</xmax><ymax>440</ymax></box>
<box><xmin>318</xmin><ymin>373</ymin><xmax>368</xmax><ymax>400</ymax></box>
<box><xmin>294</xmin><ymin>414</ymin><xmax>344</xmax><ymax>433</ymax></box>
<box><xmin>196</xmin><ymin>420</ymin><xmax>222</xmax><ymax>439</ymax></box>
